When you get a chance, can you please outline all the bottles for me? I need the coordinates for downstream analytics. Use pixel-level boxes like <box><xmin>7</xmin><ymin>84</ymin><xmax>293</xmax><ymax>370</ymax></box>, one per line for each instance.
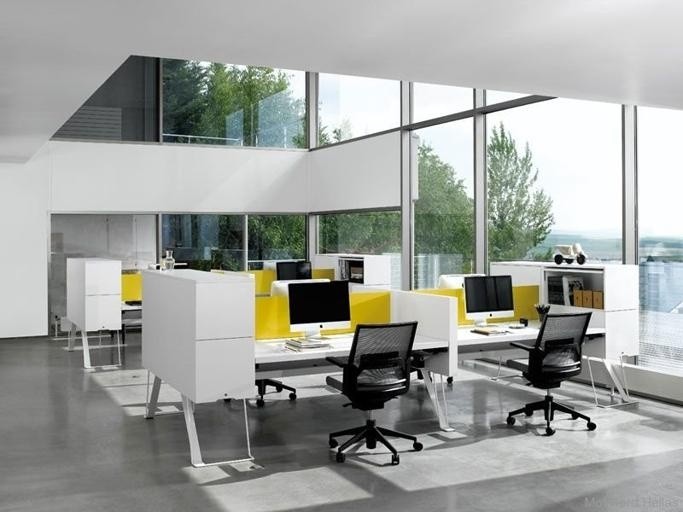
<box><xmin>163</xmin><ymin>250</ymin><xmax>175</xmax><ymax>271</ymax></box>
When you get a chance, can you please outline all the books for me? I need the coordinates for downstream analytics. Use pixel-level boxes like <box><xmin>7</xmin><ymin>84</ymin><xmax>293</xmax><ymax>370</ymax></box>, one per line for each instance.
<box><xmin>284</xmin><ymin>337</ymin><xmax>333</xmax><ymax>352</ymax></box>
<box><xmin>471</xmin><ymin>326</ymin><xmax>509</xmax><ymax>336</ymax></box>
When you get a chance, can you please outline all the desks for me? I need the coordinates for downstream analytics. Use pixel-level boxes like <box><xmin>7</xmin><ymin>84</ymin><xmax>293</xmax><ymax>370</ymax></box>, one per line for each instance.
<box><xmin>60</xmin><ymin>254</ymin><xmax>639</xmax><ymax>468</ymax></box>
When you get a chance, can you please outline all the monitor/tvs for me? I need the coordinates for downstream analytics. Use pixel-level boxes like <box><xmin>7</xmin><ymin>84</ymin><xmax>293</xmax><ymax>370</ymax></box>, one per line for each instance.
<box><xmin>275</xmin><ymin>261</ymin><xmax>312</xmax><ymax>279</ymax></box>
<box><xmin>464</xmin><ymin>274</ymin><xmax>515</xmax><ymax>333</ymax></box>
<box><xmin>287</xmin><ymin>280</ymin><xmax>352</xmax><ymax>339</ymax></box>
